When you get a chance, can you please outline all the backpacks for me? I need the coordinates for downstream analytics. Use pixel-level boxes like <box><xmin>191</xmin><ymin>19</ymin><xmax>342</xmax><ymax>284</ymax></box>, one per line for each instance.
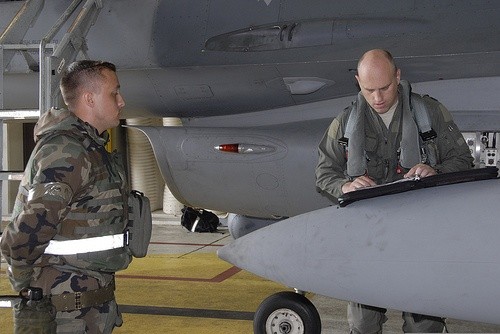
<box><xmin>180</xmin><ymin>205</ymin><xmax>222</xmax><ymax>233</ymax></box>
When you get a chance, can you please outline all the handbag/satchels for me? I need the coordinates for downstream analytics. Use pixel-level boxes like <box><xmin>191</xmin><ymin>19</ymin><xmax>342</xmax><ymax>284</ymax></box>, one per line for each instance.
<box><xmin>125</xmin><ymin>189</ymin><xmax>153</xmax><ymax>258</ymax></box>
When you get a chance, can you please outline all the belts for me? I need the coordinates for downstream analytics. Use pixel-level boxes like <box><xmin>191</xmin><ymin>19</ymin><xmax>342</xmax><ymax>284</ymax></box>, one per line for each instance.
<box><xmin>18</xmin><ymin>277</ymin><xmax>116</xmax><ymax>312</ymax></box>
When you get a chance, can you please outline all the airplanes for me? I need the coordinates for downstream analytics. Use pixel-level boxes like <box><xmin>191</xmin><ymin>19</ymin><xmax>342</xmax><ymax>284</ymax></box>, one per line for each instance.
<box><xmin>0</xmin><ymin>0</ymin><xmax>500</xmax><ymax>333</ymax></box>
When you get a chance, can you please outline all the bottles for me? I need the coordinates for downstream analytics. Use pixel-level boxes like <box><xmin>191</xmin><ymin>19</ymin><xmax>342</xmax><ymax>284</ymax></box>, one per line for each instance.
<box><xmin>14</xmin><ymin>287</ymin><xmax>57</xmax><ymax>334</ymax></box>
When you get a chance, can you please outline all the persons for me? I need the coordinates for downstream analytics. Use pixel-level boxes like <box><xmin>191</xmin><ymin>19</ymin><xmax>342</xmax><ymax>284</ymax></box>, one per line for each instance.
<box><xmin>0</xmin><ymin>59</ymin><xmax>134</xmax><ymax>334</ymax></box>
<box><xmin>314</xmin><ymin>49</ymin><xmax>473</xmax><ymax>334</ymax></box>
